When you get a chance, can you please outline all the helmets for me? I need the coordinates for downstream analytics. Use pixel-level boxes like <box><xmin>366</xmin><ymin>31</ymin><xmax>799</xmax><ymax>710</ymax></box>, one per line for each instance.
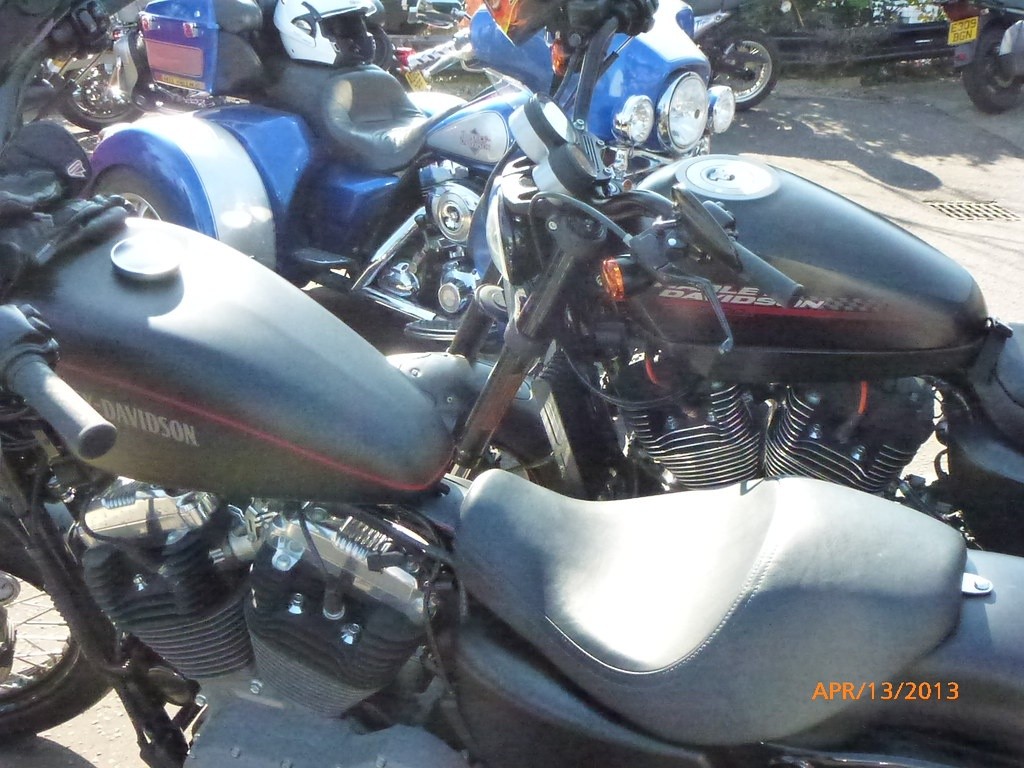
<box><xmin>273</xmin><ymin>0</ymin><xmax>393</xmax><ymax>71</ymax></box>
<box><xmin>470</xmin><ymin>0</ymin><xmax>560</xmax><ymax>100</ymax></box>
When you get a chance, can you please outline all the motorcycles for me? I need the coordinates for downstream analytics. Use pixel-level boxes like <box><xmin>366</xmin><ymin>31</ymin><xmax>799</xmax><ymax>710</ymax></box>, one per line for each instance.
<box><xmin>938</xmin><ymin>0</ymin><xmax>1023</xmax><ymax>114</ymax></box>
<box><xmin>0</xmin><ymin>0</ymin><xmax>1022</xmax><ymax>767</ymax></box>
